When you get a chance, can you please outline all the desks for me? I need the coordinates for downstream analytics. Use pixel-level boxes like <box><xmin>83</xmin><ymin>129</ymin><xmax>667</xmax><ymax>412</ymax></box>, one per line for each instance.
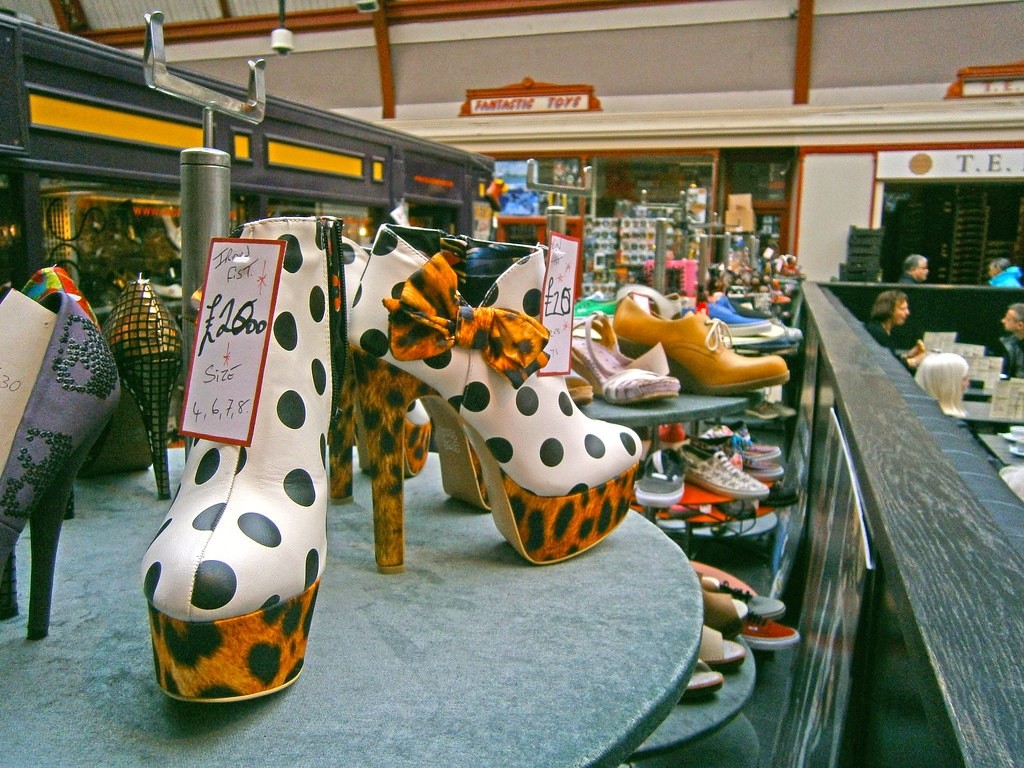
<box><xmin>969</xmin><ymin>431</ymin><xmax>1024</xmax><ymax>473</ymax></box>
<box><xmin>929</xmin><ymin>398</ymin><xmax>1023</xmax><ymax>431</ymax></box>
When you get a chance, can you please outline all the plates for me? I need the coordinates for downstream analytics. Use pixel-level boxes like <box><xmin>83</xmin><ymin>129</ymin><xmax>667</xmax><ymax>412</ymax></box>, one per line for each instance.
<box><xmin>1002</xmin><ymin>432</ymin><xmax>1022</xmax><ymax>442</ymax></box>
<box><xmin>1009</xmin><ymin>446</ymin><xmax>1024</xmax><ymax>457</ymax></box>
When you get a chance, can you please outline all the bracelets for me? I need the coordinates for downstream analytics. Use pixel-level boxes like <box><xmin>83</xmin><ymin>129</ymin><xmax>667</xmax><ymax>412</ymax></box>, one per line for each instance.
<box><xmin>910</xmin><ymin>357</ymin><xmax>917</xmax><ymax>368</ymax></box>
<box><xmin>902</xmin><ymin>350</ymin><xmax>909</xmax><ymax>358</ymax></box>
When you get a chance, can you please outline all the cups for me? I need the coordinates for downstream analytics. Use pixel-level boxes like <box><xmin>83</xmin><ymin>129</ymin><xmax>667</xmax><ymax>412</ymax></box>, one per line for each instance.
<box><xmin>1010</xmin><ymin>425</ymin><xmax>1024</xmax><ymax>438</ymax></box>
<box><xmin>1016</xmin><ymin>437</ymin><xmax>1024</xmax><ymax>451</ymax></box>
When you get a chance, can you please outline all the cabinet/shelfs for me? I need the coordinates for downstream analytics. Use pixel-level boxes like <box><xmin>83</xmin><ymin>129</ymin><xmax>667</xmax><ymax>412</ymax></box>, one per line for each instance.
<box><xmin>562</xmin><ymin>371</ymin><xmax>761</xmax><ymax>767</ymax></box>
<box><xmin>0</xmin><ymin>437</ymin><xmax>709</xmax><ymax>767</ymax></box>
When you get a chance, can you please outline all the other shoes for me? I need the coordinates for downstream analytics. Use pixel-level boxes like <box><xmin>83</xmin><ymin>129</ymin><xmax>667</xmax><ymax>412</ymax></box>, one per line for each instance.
<box><xmin>681</xmin><ymin>293</ymin><xmax>804</xmax><ymax>351</ymax></box>
<box><xmin>634</xmin><ymin>400</ymin><xmax>802</xmax><ymax>700</ymax></box>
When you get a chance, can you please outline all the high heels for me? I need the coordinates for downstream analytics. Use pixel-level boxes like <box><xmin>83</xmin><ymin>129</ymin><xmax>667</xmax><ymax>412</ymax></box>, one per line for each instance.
<box><xmin>1</xmin><ymin>215</ymin><xmax>641</xmax><ymax>704</ymax></box>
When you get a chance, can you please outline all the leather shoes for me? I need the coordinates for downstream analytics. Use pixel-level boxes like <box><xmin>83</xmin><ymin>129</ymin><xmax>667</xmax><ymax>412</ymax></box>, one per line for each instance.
<box><xmin>615</xmin><ymin>293</ymin><xmax>791</xmax><ymax>395</ymax></box>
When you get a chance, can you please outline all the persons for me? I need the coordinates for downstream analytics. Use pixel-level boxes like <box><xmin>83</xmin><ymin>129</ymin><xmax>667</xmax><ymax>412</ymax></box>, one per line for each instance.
<box><xmin>898</xmin><ymin>254</ymin><xmax>929</xmax><ymax>283</ymax></box>
<box><xmin>986</xmin><ymin>256</ymin><xmax>1024</xmax><ymax>288</ymax></box>
<box><xmin>999</xmin><ymin>302</ymin><xmax>1024</xmax><ymax>379</ymax></box>
<box><xmin>862</xmin><ymin>288</ymin><xmax>930</xmax><ymax>371</ymax></box>
<box><xmin>914</xmin><ymin>351</ymin><xmax>995</xmax><ymax>467</ymax></box>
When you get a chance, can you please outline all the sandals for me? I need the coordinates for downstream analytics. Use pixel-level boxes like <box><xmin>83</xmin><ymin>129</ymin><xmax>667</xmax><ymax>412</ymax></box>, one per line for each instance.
<box><xmin>566</xmin><ymin>310</ymin><xmax>680</xmax><ymax>407</ymax></box>
